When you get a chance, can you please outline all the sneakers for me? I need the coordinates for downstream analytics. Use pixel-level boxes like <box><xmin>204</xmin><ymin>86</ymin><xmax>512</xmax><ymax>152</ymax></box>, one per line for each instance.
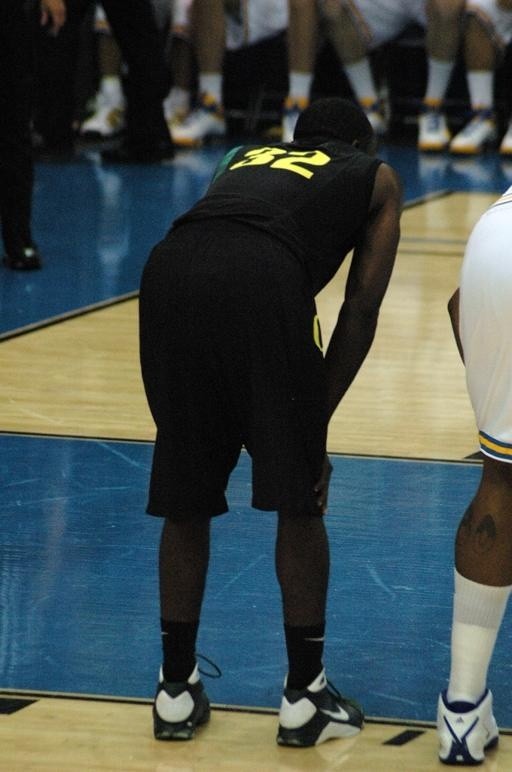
<box><xmin>276</xmin><ymin>666</ymin><xmax>368</xmax><ymax>750</ymax></box>
<box><xmin>2</xmin><ymin>241</ymin><xmax>40</xmax><ymax>274</ymax></box>
<box><xmin>80</xmin><ymin>88</ymin><xmax>228</xmax><ymax>166</ymax></box>
<box><xmin>280</xmin><ymin>91</ymin><xmax>512</xmax><ymax>158</ymax></box>
<box><xmin>152</xmin><ymin>659</ymin><xmax>212</xmax><ymax>743</ymax></box>
<box><xmin>434</xmin><ymin>686</ymin><xmax>502</xmax><ymax>766</ymax></box>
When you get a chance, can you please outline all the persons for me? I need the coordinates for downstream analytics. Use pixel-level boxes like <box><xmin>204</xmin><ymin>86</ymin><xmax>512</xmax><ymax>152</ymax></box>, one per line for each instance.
<box><xmin>138</xmin><ymin>96</ymin><xmax>402</xmax><ymax>750</ymax></box>
<box><xmin>433</xmin><ymin>184</ymin><xmax>512</xmax><ymax>766</ymax></box>
